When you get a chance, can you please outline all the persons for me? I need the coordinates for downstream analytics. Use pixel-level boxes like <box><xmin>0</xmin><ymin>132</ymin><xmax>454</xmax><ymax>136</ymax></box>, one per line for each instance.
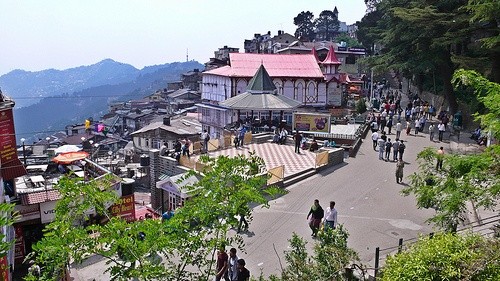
<box><xmin>28</xmin><ymin>260</ymin><xmax>40</xmax><ymax>275</ymax></box>
<box><xmin>201</xmin><ymin>129</ymin><xmax>208</xmax><ymax>151</ymax></box>
<box><xmin>469</xmin><ymin>128</ymin><xmax>481</xmax><ymax>141</ymax></box>
<box><xmin>139</xmin><ymin>208</ymin><xmax>174</xmax><ymax>239</ymax></box>
<box><xmin>235</xmin><ymin>125</ymin><xmax>246</xmax><ymax>149</ymax></box>
<box><xmin>371</xmin><ymin>80</ymin><xmax>448</xmax><ymax>162</ymax></box>
<box><xmin>436</xmin><ymin>146</ymin><xmax>444</xmax><ymax>171</ymax></box>
<box><xmin>322</xmin><ymin>201</ymin><xmax>337</xmax><ymax>230</ymax></box>
<box><xmin>302</xmin><ymin>142</ymin><xmax>309</xmax><ymax>150</ymax></box>
<box><xmin>300</xmin><ymin>132</ymin><xmax>305</xmax><ymax>144</ymax></box>
<box><xmin>271</xmin><ymin>127</ymin><xmax>288</xmax><ymax>145</ymax></box>
<box><xmin>214</xmin><ymin>244</ymin><xmax>230</xmax><ymax>281</ymax></box>
<box><xmin>160</xmin><ymin>134</ymin><xmax>190</xmax><ymax>157</ymax></box>
<box><xmin>228</xmin><ymin>248</ymin><xmax>241</xmax><ymax>281</ymax></box>
<box><xmin>236</xmin><ymin>259</ymin><xmax>250</xmax><ymax>281</ymax></box>
<box><xmin>309</xmin><ymin>138</ymin><xmax>317</xmax><ymax>152</ymax></box>
<box><xmin>307</xmin><ymin>199</ymin><xmax>324</xmax><ymax>238</ymax></box>
<box><xmin>292</xmin><ymin>129</ymin><xmax>302</xmax><ymax>154</ymax></box>
<box><xmin>395</xmin><ymin>157</ymin><xmax>404</xmax><ymax>184</ymax></box>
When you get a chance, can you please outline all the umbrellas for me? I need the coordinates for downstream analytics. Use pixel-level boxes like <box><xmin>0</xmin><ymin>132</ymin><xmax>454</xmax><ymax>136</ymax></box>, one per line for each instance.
<box><xmin>53</xmin><ymin>150</ymin><xmax>90</xmax><ymax>164</ymax></box>
<box><xmin>55</xmin><ymin>145</ymin><xmax>81</xmax><ymax>153</ymax></box>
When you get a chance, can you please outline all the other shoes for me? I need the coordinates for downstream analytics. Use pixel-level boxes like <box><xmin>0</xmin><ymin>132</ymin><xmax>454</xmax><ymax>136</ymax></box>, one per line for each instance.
<box><xmin>314</xmin><ymin>234</ymin><xmax>317</xmax><ymax>238</ymax></box>
